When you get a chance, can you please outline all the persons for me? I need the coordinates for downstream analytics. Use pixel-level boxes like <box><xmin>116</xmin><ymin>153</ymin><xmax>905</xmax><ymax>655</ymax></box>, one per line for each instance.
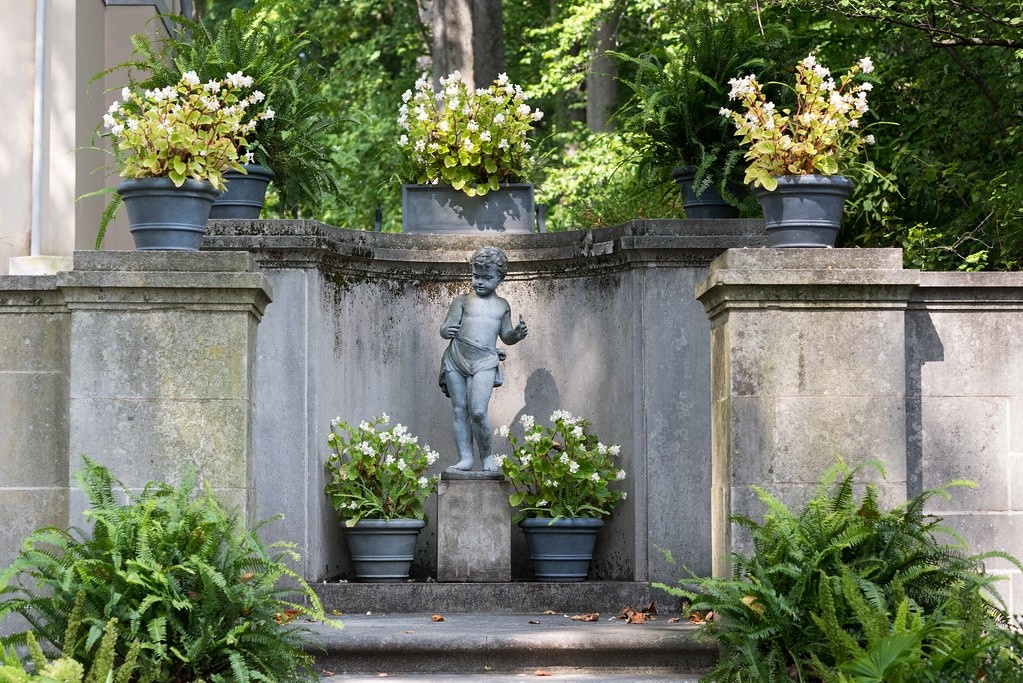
<box><xmin>439</xmin><ymin>246</ymin><xmax>527</xmax><ymax>471</ymax></box>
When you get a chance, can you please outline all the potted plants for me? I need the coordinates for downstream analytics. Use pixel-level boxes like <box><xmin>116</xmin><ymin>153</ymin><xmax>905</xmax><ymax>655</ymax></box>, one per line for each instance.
<box><xmin>599</xmin><ymin>9</ymin><xmax>796</xmax><ymax>218</ymax></box>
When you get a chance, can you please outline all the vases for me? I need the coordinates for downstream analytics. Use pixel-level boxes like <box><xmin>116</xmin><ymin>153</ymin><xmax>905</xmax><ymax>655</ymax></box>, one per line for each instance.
<box><xmin>340</xmin><ymin>518</ymin><xmax>424</xmax><ymax>583</ymax></box>
<box><xmin>403</xmin><ymin>184</ymin><xmax>534</xmax><ymax>235</ymax></box>
<box><xmin>90</xmin><ymin>1</ymin><xmax>350</xmax><ymax>220</ymax></box>
<box><xmin>519</xmin><ymin>513</ymin><xmax>605</xmax><ymax>583</ymax></box>
<box><xmin>117</xmin><ymin>179</ymin><xmax>217</xmax><ymax>252</ymax></box>
<box><xmin>755</xmin><ymin>171</ymin><xmax>857</xmax><ymax>248</ymax></box>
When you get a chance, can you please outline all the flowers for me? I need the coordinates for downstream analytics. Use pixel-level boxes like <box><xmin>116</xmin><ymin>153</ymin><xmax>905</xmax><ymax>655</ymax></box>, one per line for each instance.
<box><xmin>720</xmin><ymin>53</ymin><xmax>901</xmax><ymax>191</ymax></box>
<box><xmin>325</xmin><ymin>416</ymin><xmax>440</xmax><ymax>536</ymax></box>
<box><xmin>399</xmin><ymin>74</ymin><xmax>545</xmax><ymax>198</ymax></box>
<box><xmin>483</xmin><ymin>411</ymin><xmax>629</xmax><ymax>520</ymax></box>
<box><xmin>98</xmin><ymin>69</ymin><xmax>272</xmax><ymax>199</ymax></box>
